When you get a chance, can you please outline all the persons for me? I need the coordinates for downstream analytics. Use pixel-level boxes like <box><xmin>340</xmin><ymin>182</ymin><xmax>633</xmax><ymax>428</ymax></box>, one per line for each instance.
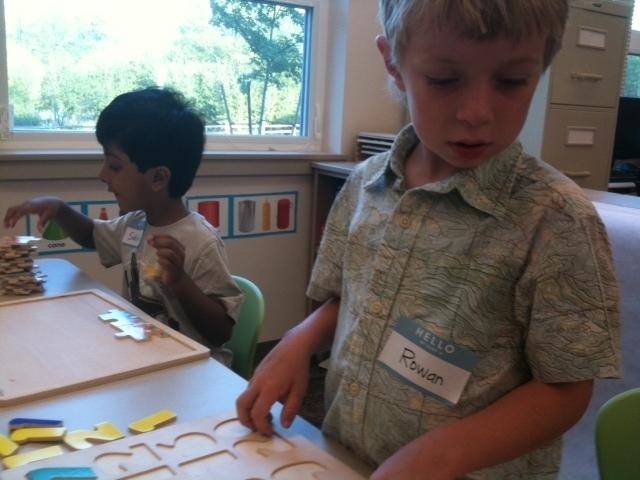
<box><xmin>0</xmin><ymin>86</ymin><xmax>247</xmax><ymax>369</ymax></box>
<box><xmin>235</xmin><ymin>0</ymin><xmax>624</xmax><ymax>480</ymax></box>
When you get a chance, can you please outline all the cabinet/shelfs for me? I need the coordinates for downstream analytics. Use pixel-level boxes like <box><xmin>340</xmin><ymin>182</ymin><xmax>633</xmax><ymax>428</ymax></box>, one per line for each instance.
<box><xmin>515</xmin><ymin>0</ymin><xmax>636</xmax><ymax>193</ymax></box>
